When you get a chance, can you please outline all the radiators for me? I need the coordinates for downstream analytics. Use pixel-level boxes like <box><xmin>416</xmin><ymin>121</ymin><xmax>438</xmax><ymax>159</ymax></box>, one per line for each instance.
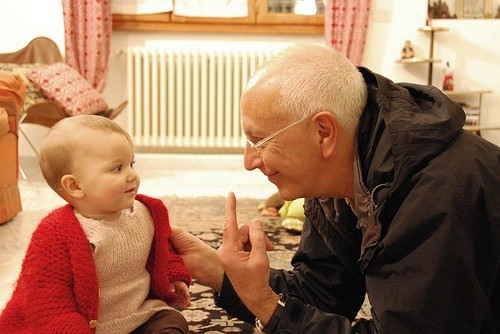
<box><xmin>124</xmin><ymin>48</ymin><xmax>272</xmax><ymax>153</ymax></box>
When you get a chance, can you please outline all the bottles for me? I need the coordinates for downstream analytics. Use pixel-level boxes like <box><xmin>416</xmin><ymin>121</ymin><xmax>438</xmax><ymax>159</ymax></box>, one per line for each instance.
<box><xmin>443</xmin><ymin>61</ymin><xmax>454</xmax><ymax>90</ymax></box>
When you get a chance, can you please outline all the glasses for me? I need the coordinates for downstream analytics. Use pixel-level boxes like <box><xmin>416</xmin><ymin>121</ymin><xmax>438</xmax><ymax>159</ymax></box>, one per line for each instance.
<box><xmin>246</xmin><ymin>114</ymin><xmax>315</xmax><ymax>154</ymax></box>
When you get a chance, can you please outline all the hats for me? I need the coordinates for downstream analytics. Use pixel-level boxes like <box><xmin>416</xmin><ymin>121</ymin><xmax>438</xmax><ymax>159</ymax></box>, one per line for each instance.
<box><xmin>281</xmin><ymin>198</ymin><xmax>306</xmax><ymax>231</ymax></box>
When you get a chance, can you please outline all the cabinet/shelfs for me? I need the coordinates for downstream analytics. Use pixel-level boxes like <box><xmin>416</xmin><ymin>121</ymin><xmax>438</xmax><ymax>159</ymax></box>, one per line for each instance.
<box><xmin>395</xmin><ymin>25</ymin><xmax>492</xmax><ymax>136</ymax></box>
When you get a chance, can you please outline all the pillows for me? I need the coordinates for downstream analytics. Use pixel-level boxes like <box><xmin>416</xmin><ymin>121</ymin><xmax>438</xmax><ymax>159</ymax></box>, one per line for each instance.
<box><xmin>26</xmin><ymin>60</ymin><xmax>109</xmax><ymax>116</ymax></box>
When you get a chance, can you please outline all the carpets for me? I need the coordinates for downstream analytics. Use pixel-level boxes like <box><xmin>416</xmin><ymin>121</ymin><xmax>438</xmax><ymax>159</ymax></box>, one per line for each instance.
<box><xmin>0</xmin><ymin>195</ymin><xmax>372</xmax><ymax>334</ymax></box>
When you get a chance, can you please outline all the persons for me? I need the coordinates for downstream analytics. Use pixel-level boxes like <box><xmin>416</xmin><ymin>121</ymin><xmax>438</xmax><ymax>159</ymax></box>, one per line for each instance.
<box><xmin>171</xmin><ymin>41</ymin><xmax>500</xmax><ymax>333</ymax></box>
<box><xmin>1</xmin><ymin>115</ymin><xmax>190</xmax><ymax>334</ymax></box>
